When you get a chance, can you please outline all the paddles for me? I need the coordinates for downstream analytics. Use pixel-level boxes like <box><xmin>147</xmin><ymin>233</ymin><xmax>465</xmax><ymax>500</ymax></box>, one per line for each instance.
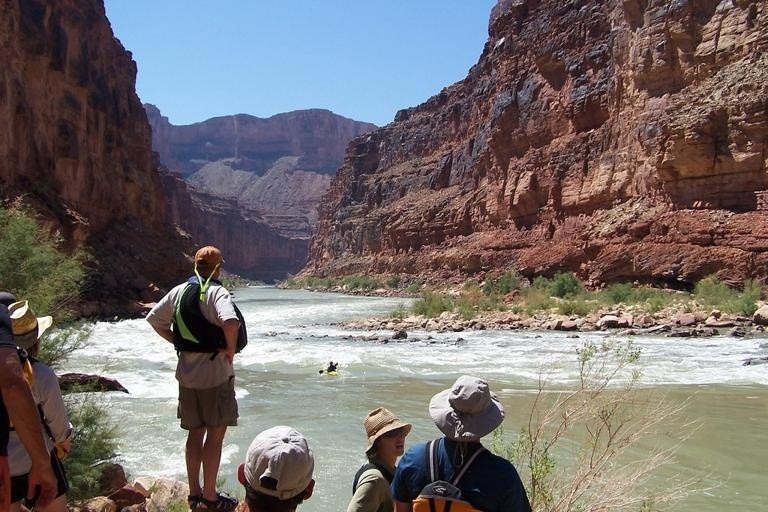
<box><xmin>319</xmin><ymin>363</ymin><xmax>338</xmax><ymax>374</ymax></box>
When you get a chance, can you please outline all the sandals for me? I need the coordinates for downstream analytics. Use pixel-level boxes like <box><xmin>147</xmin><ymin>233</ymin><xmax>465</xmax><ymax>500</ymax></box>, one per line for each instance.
<box><xmin>187</xmin><ymin>490</ymin><xmax>239</xmax><ymax>511</ymax></box>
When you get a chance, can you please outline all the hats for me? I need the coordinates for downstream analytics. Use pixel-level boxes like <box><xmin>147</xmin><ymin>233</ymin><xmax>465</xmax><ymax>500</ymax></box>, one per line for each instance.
<box><xmin>245</xmin><ymin>425</ymin><xmax>314</xmax><ymax>499</ymax></box>
<box><xmin>365</xmin><ymin>407</ymin><xmax>412</xmax><ymax>452</ymax></box>
<box><xmin>195</xmin><ymin>246</ymin><xmax>225</xmax><ymax>267</ymax></box>
<box><xmin>7</xmin><ymin>300</ymin><xmax>53</xmax><ymax>349</ymax></box>
<box><xmin>429</xmin><ymin>376</ymin><xmax>505</xmax><ymax>440</ymax></box>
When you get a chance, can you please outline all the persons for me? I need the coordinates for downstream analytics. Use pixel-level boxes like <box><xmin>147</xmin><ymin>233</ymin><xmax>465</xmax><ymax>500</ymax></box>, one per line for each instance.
<box><xmin>390</xmin><ymin>374</ymin><xmax>533</xmax><ymax>512</ymax></box>
<box><xmin>346</xmin><ymin>407</ymin><xmax>411</xmax><ymax>512</ymax></box>
<box><xmin>6</xmin><ymin>300</ymin><xmax>74</xmax><ymax>512</ymax></box>
<box><xmin>237</xmin><ymin>425</ymin><xmax>315</xmax><ymax>512</ymax></box>
<box><xmin>0</xmin><ymin>291</ymin><xmax>17</xmax><ymax>306</ymax></box>
<box><xmin>146</xmin><ymin>245</ymin><xmax>239</xmax><ymax>512</ymax></box>
<box><xmin>325</xmin><ymin>361</ymin><xmax>337</xmax><ymax>372</ymax></box>
<box><xmin>0</xmin><ymin>304</ymin><xmax>58</xmax><ymax>512</ymax></box>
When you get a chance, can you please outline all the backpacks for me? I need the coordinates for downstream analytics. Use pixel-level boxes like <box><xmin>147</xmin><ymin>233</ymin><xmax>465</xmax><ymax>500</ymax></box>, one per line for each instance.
<box><xmin>412</xmin><ymin>438</ymin><xmax>486</xmax><ymax>512</ymax></box>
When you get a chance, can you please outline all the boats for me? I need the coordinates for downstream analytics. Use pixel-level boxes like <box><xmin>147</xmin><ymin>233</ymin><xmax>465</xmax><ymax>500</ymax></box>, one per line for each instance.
<box><xmin>324</xmin><ymin>370</ymin><xmax>338</xmax><ymax>376</ymax></box>
